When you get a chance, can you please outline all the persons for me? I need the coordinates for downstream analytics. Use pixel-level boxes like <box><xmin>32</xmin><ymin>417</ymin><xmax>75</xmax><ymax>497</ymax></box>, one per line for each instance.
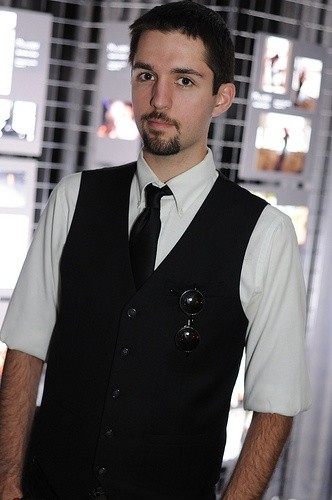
<box><xmin>0</xmin><ymin>0</ymin><xmax>305</xmax><ymax>500</ymax></box>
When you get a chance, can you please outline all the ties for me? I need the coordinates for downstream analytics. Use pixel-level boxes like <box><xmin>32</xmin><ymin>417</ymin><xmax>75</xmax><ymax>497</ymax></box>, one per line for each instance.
<box><xmin>130</xmin><ymin>184</ymin><xmax>174</xmax><ymax>291</ymax></box>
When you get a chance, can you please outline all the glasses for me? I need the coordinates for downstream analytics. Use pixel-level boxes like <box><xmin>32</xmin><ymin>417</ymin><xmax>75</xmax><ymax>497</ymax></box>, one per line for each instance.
<box><xmin>174</xmin><ymin>287</ymin><xmax>207</xmax><ymax>354</ymax></box>
